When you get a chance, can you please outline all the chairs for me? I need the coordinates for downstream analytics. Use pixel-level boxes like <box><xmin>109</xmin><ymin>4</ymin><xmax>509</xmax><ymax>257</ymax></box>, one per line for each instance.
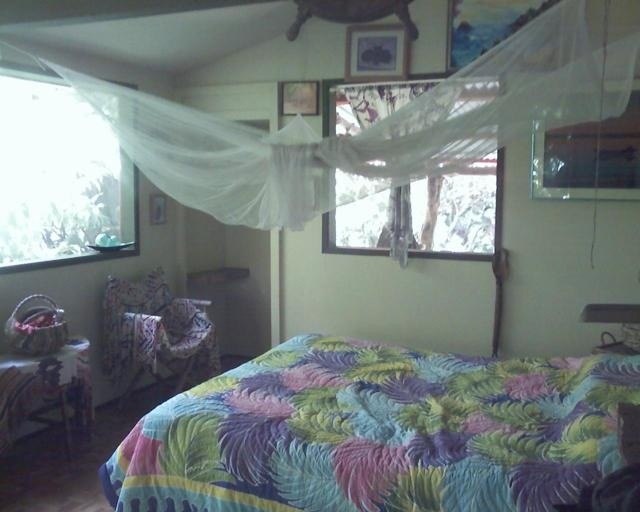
<box><xmin>108</xmin><ymin>273</ymin><xmax>213</xmax><ymax>417</ymax></box>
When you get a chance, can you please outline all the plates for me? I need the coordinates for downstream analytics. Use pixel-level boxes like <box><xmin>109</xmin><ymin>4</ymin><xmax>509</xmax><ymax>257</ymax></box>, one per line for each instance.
<box><xmin>86</xmin><ymin>241</ymin><xmax>136</xmax><ymax>251</ymax></box>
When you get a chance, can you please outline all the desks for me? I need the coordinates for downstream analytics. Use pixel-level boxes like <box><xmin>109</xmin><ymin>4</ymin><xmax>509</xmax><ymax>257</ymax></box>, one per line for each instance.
<box><xmin>0</xmin><ymin>338</ymin><xmax>92</xmax><ymax>487</ymax></box>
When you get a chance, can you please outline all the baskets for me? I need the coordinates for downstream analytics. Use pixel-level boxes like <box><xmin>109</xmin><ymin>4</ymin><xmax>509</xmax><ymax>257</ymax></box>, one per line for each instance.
<box><xmin>4</xmin><ymin>294</ymin><xmax>68</xmax><ymax>355</ymax></box>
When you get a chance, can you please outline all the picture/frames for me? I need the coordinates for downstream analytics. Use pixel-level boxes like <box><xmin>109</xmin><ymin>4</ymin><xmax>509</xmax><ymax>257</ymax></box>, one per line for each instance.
<box><xmin>533</xmin><ymin>75</ymin><xmax>640</xmax><ymax>198</ymax></box>
<box><xmin>278</xmin><ymin>81</ymin><xmax>319</xmax><ymax>117</ymax></box>
<box><xmin>446</xmin><ymin>0</ymin><xmax>564</xmax><ymax>78</ymax></box>
<box><xmin>345</xmin><ymin>23</ymin><xmax>411</xmax><ymax>81</ymax></box>
<box><xmin>150</xmin><ymin>193</ymin><xmax>169</xmax><ymax>226</ymax></box>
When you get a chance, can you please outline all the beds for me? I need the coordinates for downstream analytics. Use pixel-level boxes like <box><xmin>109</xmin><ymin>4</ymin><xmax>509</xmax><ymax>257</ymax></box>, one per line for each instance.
<box><xmin>97</xmin><ymin>335</ymin><xmax>640</xmax><ymax>512</ymax></box>
